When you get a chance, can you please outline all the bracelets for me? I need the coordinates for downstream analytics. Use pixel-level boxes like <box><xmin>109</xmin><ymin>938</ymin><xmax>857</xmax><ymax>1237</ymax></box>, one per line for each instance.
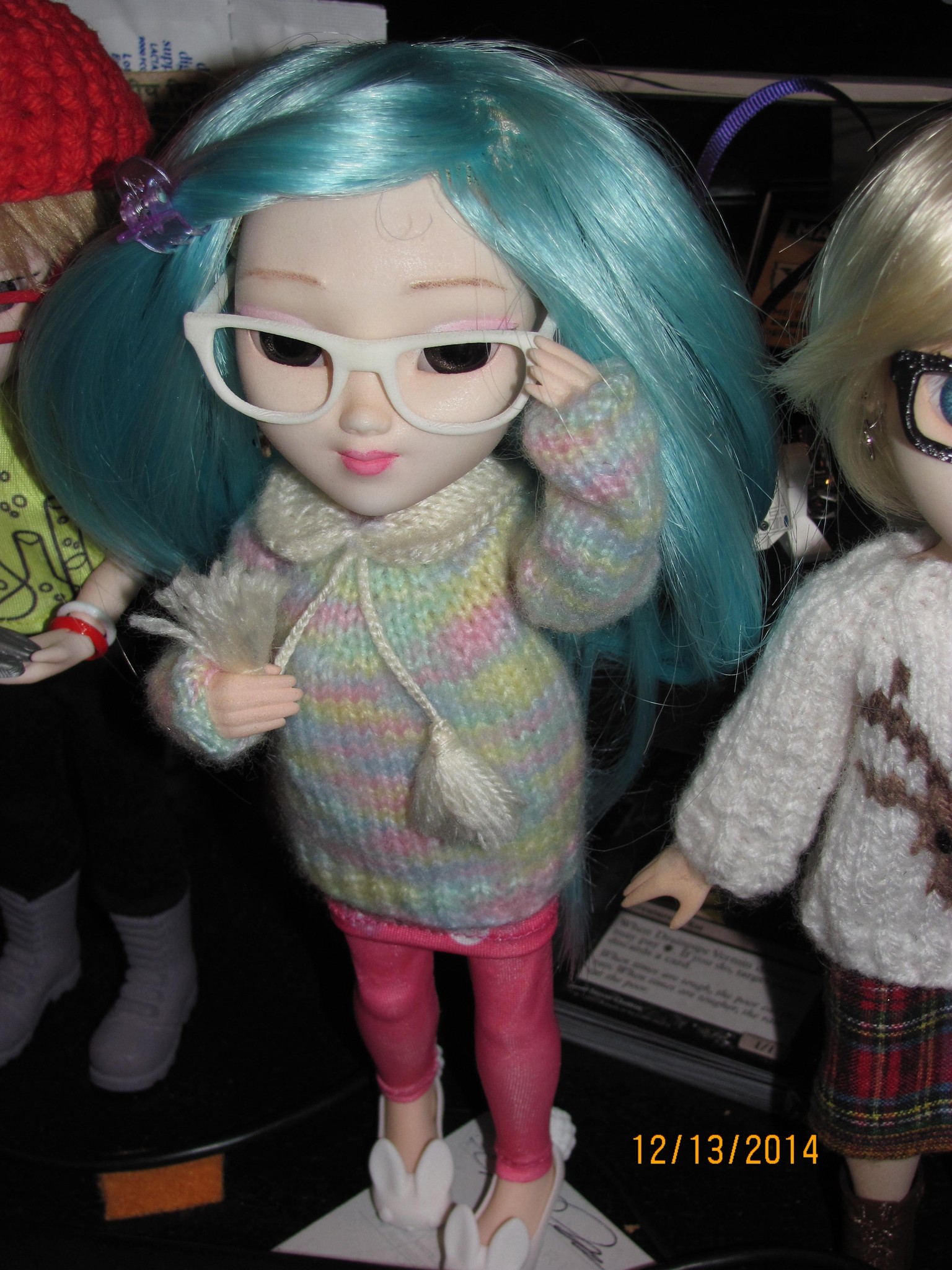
<box><xmin>58</xmin><ymin>601</ymin><xmax>116</xmax><ymax>647</ymax></box>
<box><xmin>48</xmin><ymin>617</ymin><xmax>109</xmax><ymax>661</ymax></box>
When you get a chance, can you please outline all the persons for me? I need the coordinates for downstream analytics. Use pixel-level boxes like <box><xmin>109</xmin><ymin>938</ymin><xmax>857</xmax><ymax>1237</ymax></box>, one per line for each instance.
<box><xmin>10</xmin><ymin>40</ymin><xmax>783</xmax><ymax>1270</ymax></box>
<box><xmin>0</xmin><ymin>0</ymin><xmax>201</xmax><ymax>1093</ymax></box>
<box><xmin>621</xmin><ymin>115</ymin><xmax>952</xmax><ymax>1270</ymax></box>
